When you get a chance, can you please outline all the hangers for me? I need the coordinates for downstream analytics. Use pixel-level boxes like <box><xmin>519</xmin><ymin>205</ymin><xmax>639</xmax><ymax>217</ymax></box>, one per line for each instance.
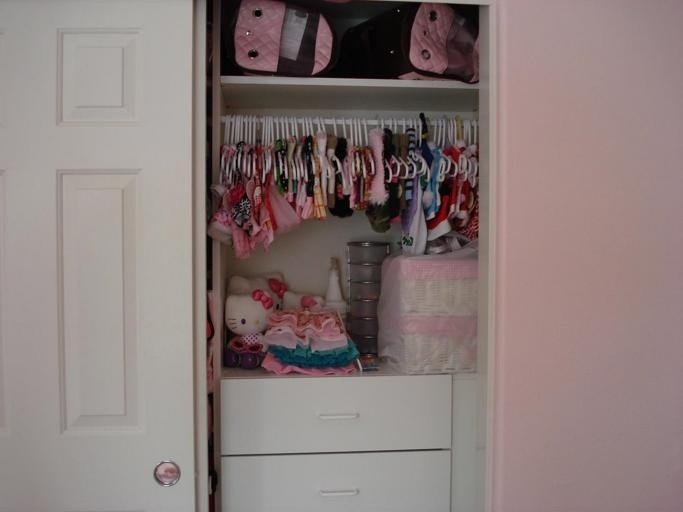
<box><xmin>219</xmin><ymin>115</ymin><xmax>480</xmax><ymax>188</ymax></box>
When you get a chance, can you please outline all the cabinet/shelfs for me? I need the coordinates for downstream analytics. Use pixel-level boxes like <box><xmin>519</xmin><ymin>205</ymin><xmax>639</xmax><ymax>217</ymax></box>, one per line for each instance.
<box><xmin>211</xmin><ymin>0</ymin><xmax>497</xmax><ymax>512</ymax></box>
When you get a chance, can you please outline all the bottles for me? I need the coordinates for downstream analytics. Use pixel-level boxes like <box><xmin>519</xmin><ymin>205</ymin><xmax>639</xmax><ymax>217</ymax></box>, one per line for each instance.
<box><xmin>325</xmin><ymin>257</ymin><xmax>348</xmax><ymax>319</ymax></box>
